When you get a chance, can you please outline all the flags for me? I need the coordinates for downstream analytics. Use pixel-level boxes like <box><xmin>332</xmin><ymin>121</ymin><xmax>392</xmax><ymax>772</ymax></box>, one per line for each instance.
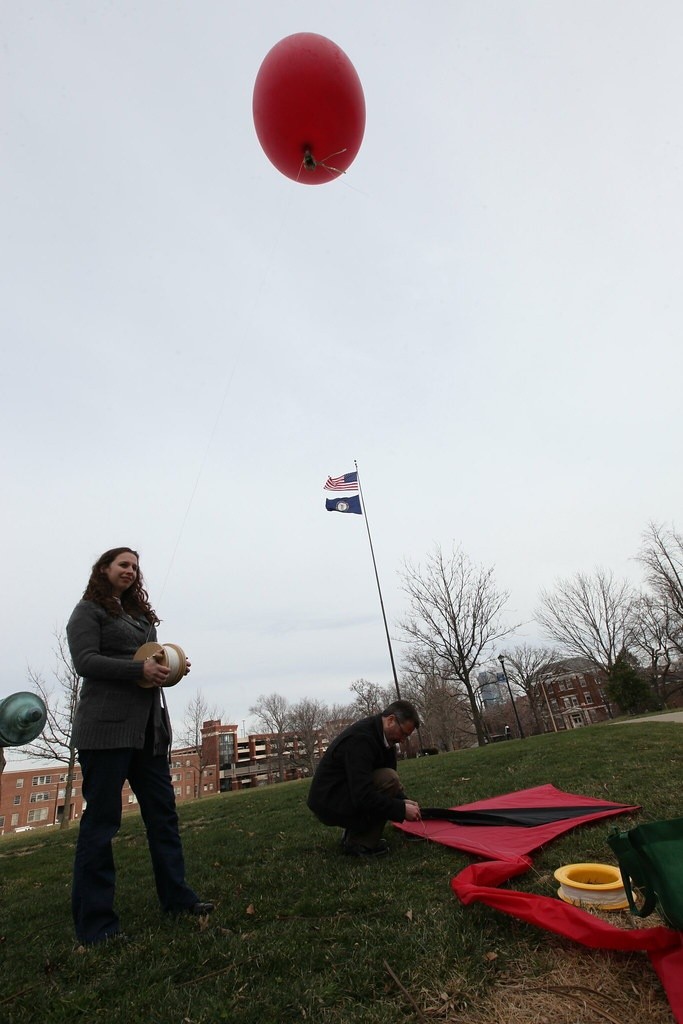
<box><xmin>324</xmin><ymin>471</ymin><xmax>363</xmax><ymax>514</ymax></box>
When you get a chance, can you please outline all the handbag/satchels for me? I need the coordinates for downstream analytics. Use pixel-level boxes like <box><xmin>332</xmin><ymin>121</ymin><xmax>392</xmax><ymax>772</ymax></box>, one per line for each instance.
<box><xmin>608</xmin><ymin>817</ymin><xmax>683</xmax><ymax>931</ymax></box>
<box><xmin>162</xmin><ymin>707</ymin><xmax>172</xmax><ymax>745</ymax></box>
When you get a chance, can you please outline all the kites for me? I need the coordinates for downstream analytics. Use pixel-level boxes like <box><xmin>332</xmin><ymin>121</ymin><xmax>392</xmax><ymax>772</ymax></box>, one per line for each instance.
<box><xmin>392</xmin><ymin>783</ymin><xmax>683</xmax><ymax>1024</ymax></box>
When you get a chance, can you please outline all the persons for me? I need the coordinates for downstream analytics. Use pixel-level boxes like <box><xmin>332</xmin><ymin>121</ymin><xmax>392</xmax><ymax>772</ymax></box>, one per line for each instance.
<box><xmin>306</xmin><ymin>699</ymin><xmax>421</xmax><ymax>858</ymax></box>
<box><xmin>504</xmin><ymin>723</ymin><xmax>513</xmax><ymax>740</ymax></box>
<box><xmin>65</xmin><ymin>547</ymin><xmax>216</xmax><ymax>947</ymax></box>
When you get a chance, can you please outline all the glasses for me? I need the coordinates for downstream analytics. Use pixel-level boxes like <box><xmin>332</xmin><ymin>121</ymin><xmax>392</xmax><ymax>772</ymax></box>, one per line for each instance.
<box><xmin>394</xmin><ymin>714</ymin><xmax>408</xmax><ymax>739</ymax></box>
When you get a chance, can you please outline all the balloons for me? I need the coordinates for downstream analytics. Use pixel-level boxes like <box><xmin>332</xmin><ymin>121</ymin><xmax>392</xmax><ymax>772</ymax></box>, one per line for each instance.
<box><xmin>252</xmin><ymin>33</ymin><xmax>367</xmax><ymax>184</ymax></box>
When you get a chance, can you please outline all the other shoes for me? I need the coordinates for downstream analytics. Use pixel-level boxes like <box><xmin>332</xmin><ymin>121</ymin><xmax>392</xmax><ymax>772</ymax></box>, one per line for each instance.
<box><xmin>189</xmin><ymin>902</ymin><xmax>213</xmax><ymax>914</ymax></box>
